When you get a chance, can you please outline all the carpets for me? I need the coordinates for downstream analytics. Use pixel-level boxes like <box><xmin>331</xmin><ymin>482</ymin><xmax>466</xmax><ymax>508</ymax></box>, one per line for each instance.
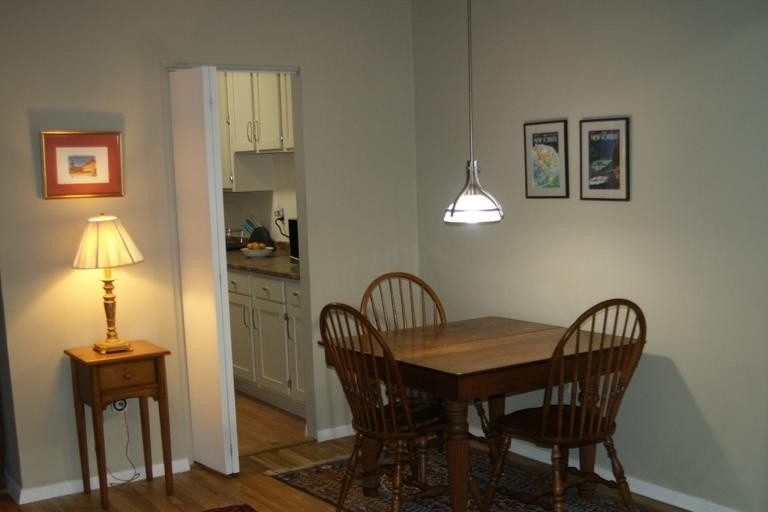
<box><xmin>203</xmin><ymin>503</ymin><xmax>258</xmax><ymax>512</ymax></box>
<box><xmin>263</xmin><ymin>440</ymin><xmax>661</xmax><ymax>512</ymax></box>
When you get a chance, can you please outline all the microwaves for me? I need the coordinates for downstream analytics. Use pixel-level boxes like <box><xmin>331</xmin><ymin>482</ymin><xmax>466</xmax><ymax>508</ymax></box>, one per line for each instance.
<box><xmin>288</xmin><ymin>219</ymin><xmax>299</xmax><ymax>263</ymax></box>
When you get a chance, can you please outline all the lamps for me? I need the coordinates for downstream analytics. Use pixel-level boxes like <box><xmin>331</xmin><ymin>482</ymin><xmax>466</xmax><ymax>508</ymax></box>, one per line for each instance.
<box><xmin>442</xmin><ymin>0</ymin><xmax>505</xmax><ymax>225</ymax></box>
<box><xmin>71</xmin><ymin>213</ymin><xmax>145</xmax><ymax>355</ymax></box>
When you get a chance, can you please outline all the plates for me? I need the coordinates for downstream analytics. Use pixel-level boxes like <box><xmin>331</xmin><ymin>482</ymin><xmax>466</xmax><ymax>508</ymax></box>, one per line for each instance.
<box><xmin>241</xmin><ymin>248</ymin><xmax>274</xmax><ymax>258</ymax></box>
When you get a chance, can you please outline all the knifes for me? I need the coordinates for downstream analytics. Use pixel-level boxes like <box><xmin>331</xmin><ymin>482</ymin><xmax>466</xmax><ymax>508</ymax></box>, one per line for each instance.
<box><xmin>243</xmin><ymin>216</ymin><xmax>259</xmax><ymax>234</ymax></box>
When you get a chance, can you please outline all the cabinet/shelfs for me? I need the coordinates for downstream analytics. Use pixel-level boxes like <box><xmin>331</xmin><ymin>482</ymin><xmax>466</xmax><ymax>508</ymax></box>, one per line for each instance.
<box><xmin>217</xmin><ymin>69</ymin><xmax>297</xmax><ymax>193</ymax></box>
<box><xmin>286</xmin><ymin>279</ymin><xmax>308</xmax><ymax>421</ymax></box>
<box><xmin>228</xmin><ymin>264</ymin><xmax>286</xmax><ymax>414</ymax></box>
<box><xmin>63</xmin><ymin>339</ymin><xmax>173</xmax><ymax>511</ymax></box>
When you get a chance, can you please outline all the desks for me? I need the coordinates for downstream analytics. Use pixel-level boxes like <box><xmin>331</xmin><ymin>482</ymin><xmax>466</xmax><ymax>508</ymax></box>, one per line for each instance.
<box><xmin>317</xmin><ymin>314</ymin><xmax>646</xmax><ymax>512</ymax></box>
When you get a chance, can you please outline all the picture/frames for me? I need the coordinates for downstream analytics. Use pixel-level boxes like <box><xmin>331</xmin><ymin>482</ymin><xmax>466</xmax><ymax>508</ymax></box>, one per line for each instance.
<box><xmin>41</xmin><ymin>131</ymin><xmax>125</xmax><ymax>200</ymax></box>
<box><xmin>579</xmin><ymin>117</ymin><xmax>630</xmax><ymax>201</ymax></box>
<box><xmin>524</xmin><ymin>120</ymin><xmax>569</xmax><ymax>198</ymax></box>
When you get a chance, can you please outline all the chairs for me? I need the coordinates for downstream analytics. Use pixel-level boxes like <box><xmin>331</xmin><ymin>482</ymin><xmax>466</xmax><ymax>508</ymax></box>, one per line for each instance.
<box><xmin>320</xmin><ymin>301</ymin><xmax>485</xmax><ymax>512</ymax></box>
<box><xmin>482</xmin><ymin>299</ymin><xmax>645</xmax><ymax>512</ymax></box>
<box><xmin>357</xmin><ymin>273</ymin><xmax>448</xmax><ymax>403</ymax></box>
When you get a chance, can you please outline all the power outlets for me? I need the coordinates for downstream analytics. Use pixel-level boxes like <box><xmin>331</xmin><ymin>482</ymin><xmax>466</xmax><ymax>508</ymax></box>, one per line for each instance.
<box><xmin>280</xmin><ymin>208</ymin><xmax>286</xmax><ymax>224</ymax></box>
<box><xmin>274</xmin><ymin>211</ymin><xmax>280</xmax><ymax>218</ymax></box>
<box><xmin>111</xmin><ymin>399</ymin><xmax>128</xmax><ymax>414</ymax></box>
